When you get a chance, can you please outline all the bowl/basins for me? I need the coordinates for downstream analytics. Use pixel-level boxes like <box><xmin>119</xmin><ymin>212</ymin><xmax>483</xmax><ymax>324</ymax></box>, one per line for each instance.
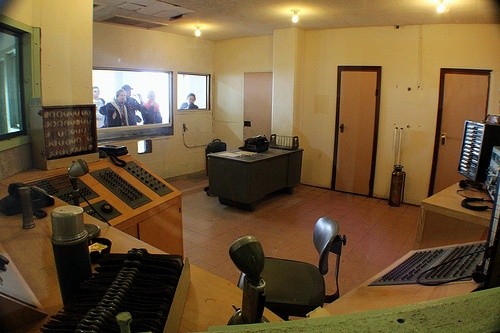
<box><xmin>88</xmin><ymin>237</ymin><xmax>113</xmax><ymax>262</ymax></box>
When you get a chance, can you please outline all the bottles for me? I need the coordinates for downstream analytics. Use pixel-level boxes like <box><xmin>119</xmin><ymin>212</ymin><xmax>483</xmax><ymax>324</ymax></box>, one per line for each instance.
<box><xmin>50</xmin><ymin>205</ymin><xmax>92</xmax><ymax>307</ymax></box>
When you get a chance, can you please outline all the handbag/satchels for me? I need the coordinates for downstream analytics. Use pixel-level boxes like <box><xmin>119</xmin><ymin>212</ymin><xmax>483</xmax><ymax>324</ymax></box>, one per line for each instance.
<box><xmin>98</xmin><ymin>143</ymin><xmax>128</xmax><ymax>167</ymax></box>
<box><xmin>207</xmin><ymin>139</ymin><xmax>226</xmax><ymax>152</ymax></box>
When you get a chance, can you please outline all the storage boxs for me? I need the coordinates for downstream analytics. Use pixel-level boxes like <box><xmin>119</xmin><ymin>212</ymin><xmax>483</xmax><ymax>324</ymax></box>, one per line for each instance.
<box><xmin>457</xmin><ymin>119</ymin><xmax>500</xmax><ymax>182</ymax></box>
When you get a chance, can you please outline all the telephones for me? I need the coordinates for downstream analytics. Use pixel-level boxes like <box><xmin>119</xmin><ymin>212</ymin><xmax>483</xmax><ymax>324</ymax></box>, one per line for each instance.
<box><xmin>0</xmin><ymin>182</ymin><xmax>55</xmax><ymax>216</ymax></box>
<box><xmin>97</xmin><ymin>144</ymin><xmax>128</xmax><ymax>156</ymax></box>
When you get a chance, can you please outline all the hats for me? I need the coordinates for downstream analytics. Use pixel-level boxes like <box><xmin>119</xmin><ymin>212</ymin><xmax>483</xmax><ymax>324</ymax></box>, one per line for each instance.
<box><xmin>122</xmin><ymin>85</ymin><xmax>133</xmax><ymax>92</ymax></box>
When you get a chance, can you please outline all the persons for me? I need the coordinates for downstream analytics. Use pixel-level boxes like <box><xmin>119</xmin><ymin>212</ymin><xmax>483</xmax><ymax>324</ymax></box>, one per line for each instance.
<box><xmin>121</xmin><ymin>84</ymin><xmax>145</xmax><ymax>125</ymax></box>
<box><xmin>100</xmin><ymin>88</ymin><xmax>131</xmax><ymax>126</ymax></box>
<box><xmin>142</xmin><ymin>90</ymin><xmax>163</xmax><ymax>124</ymax></box>
<box><xmin>181</xmin><ymin>93</ymin><xmax>199</xmax><ymax>109</ymax></box>
<box><xmin>93</xmin><ymin>86</ymin><xmax>106</xmax><ymax>127</ymax></box>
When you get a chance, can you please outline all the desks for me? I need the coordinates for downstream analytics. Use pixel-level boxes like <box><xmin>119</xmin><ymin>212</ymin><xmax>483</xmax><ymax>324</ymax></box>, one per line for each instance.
<box><xmin>0</xmin><ymin>184</ymin><xmax>285</xmax><ymax>333</ymax></box>
<box><xmin>207</xmin><ymin>144</ymin><xmax>304</xmax><ymax>211</ymax></box>
<box><xmin>413</xmin><ymin>179</ymin><xmax>494</xmax><ymax>251</ymax></box>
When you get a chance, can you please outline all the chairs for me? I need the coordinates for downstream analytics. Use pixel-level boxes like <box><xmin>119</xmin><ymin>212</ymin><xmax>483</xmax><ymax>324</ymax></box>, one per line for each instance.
<box><xmin>237</xmin><ymin>216</ymin><xmax>346</xmax><ymax>322</ymax></box>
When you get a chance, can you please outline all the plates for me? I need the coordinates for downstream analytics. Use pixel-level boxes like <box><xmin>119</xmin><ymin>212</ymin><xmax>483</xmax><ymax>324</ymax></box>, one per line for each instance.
<box><xmin>84</xmin><ymin>224</ymin><xmax>100</xmax><ymax>239</ymax></box>
